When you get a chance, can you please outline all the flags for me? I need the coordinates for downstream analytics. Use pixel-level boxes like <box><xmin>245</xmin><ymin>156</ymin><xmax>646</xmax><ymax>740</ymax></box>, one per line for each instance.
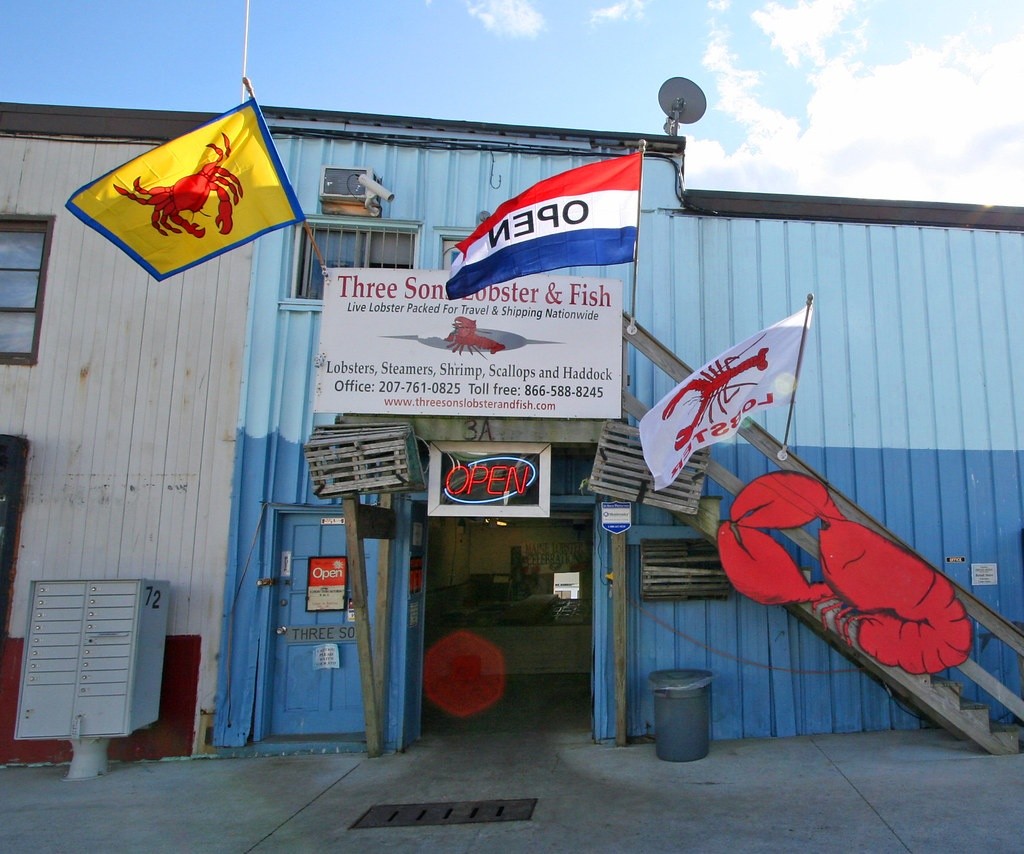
<box><xmin>445</xmin><ymin>151</ymin><xmax>644</xmax><ymax>301</ymax></box>
<box><xmin>638</xmin><ymin>300</ymin><xmax>813</xmax><ymax>492</ymax></box>
<box><xmin>63</xmin><ymin>95</ymin><xmax>305</xmax><ymax>282</ymax></box>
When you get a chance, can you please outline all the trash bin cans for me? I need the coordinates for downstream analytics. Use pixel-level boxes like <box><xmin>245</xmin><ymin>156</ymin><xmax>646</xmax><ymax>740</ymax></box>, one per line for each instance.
<box><xmin>647</xmin><ymin>670</ymin><xmax>715</xmax><ymax>763</ymax></box>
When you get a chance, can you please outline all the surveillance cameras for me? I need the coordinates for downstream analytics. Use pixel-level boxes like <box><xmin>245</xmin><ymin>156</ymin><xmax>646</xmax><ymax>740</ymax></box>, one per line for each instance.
<box><xmin>358</xmin><ymin>174</ymin><xmax>395</xmax><ymax>203</ymax></box>
